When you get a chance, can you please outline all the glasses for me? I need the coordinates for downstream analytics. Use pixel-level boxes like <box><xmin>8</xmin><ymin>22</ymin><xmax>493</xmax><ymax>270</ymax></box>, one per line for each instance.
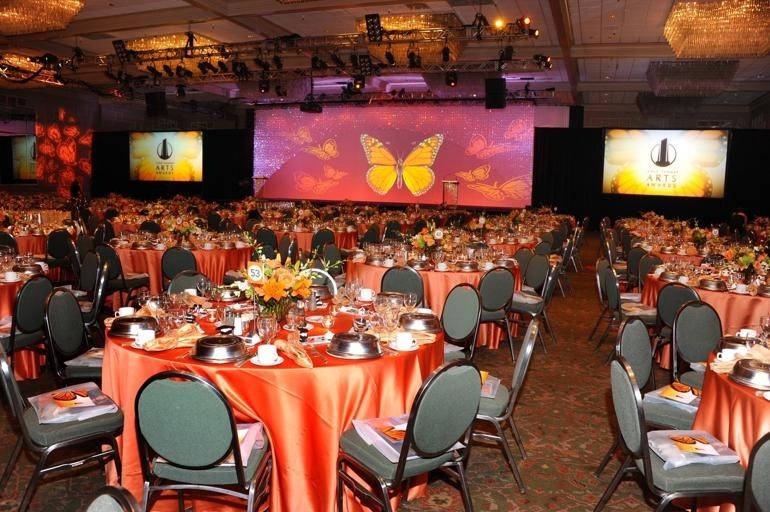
<box><xmin>633</xmin><ymin>1</ymin><xmax>769</xmax><ymax>129</ymax></box>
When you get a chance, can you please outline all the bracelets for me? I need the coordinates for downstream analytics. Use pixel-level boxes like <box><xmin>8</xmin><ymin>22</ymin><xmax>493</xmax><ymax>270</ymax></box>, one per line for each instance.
<box><xmin>719</xmin><ymin>335</ymin><xmax>762</xmax><ymax>350</ymax></box>
<box><xmin>730</xmin><ymin>358</ymin><xmax>770</xmax><ymax>391</ymax></box>
<box><xmin>653</xmin><ymin>257</ymin><xmax>770</xmax><ymax>299</ymax></box>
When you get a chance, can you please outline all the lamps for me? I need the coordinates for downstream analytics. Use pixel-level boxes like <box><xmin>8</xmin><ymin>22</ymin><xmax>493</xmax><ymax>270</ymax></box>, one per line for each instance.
<box><xmin>0</xmin><ymin>0</ymin><xmax>563</xmax><ymax>115</ymax></box>
<box><xmin>633</xmin><ymin>1</ymin><xmax>769</xmax><ymax>129</ymax></box>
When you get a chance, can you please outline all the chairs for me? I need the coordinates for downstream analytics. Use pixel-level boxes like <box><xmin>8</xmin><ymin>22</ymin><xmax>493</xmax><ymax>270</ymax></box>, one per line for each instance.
<box><xmin>0</xmin><ymin>192</ymin><xmax>583</xmax><ymax>374</ymax></box>
<box><xmin>0</xmin><ymin>346</ymin><xmax>124</xmax><ymax>510</ymax></box>
<box><xmin>337</xmin><ymin>358</ymin><xmax>483</xmax><ymax>510</ymax></box>
<box><xmin>461</xmin><ymin>317</ymin><xmax>530</xmax><ymax>497</ymax></box>
<box><xmin>593</xmin><ymin>210</ymin><xmax>769</xmax><ymax>510</ymax></box>
<box><xmin>134</xmin><ymin>370</ymin><xmax>274</xmax><ymax>510</ymax></box>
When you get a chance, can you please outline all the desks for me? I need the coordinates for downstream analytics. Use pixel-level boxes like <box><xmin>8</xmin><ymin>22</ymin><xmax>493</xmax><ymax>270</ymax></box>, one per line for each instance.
<box><xmin>101</xmin><ymin>291</ymin><xmax>445</xmax><ymax>510</ymax></box>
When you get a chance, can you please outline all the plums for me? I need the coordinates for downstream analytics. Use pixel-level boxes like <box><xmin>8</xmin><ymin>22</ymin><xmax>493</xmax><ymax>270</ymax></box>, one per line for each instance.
<box><xmin>300</xmin><ymin>101</ymin><xmax>322</xmax><ymax>114</ymax></box>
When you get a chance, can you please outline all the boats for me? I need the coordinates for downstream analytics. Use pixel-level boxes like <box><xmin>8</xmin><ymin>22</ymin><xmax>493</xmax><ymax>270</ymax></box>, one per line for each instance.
<box><xmin>144</xmin><ymin>91</ymin><xmax>169</xmax><ymax>119</ymax></box>
<box><xmin>484</xmin><ymin>77</ymin><xmax>506</xmax><ymax>109</ymax></box>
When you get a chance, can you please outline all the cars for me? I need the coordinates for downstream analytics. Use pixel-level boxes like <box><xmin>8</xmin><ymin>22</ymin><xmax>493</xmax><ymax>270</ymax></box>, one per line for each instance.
<box><xmin>736</xmin><ymin>328</ymin><xmax>756</xmax><ymax>337</ymax></box>
<box><xmin>0</xmin><ymin>247</ymin><xmax>48</xmax><ymax>283</ymax></box>
<box><xmin>352</xmin><ymin>256</ymin><xmax>516</xmax><ymax>273</ymax></box>
<box><xmin>1</xmin><ymin>209</ymin><xmax>56</xmax><ymax>237</ymax></box>
<box><xmin>717</xmin><ymin>347</ymin><xmax>737</xmax><ymax>361</ymax></box>
<box><xmin>746</xmin><ymin>332</ymin><xmax>760</xmax><ymax>349</ymax></box>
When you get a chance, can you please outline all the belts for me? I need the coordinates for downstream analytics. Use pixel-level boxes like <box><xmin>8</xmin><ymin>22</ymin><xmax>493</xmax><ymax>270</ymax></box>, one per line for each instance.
<box><xmin>760</xmin><ymin>315</ymin><xmax>770</xmax><ymax>344</ymax></box>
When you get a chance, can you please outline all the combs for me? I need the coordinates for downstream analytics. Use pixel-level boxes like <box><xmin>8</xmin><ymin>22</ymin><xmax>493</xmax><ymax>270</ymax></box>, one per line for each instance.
<box><xmin>360</xmin><ymin>132</ymin><xmax>443</xmax><ymax>197</ymax></box>
<box><xmin>308</xmin><ymin>137</ymin><xmax>340</xmax><ymax>161</ymax></box>
<box><xmin>454</xmin><ymin>165</ymin><xmax>491</xmax><ymax>183</ymax></box>
<box><xmin>466</xmin><ymin>175</ymin><xmax>531</xmax><ymax>201</ymax></box>
<box><xmin>323</xmin><ymin>164</ymin><xmax>347</xmax><ymax>181</ymax></box>
<box><xmin>294</xmin><ymin>170</ymin><xmax>339</xmax><ymax>195</ymax></box>
<box><xmin>464</xmin><ymin>134</ymin><xmax>498</xmax><ymax>160</ymax></box>
